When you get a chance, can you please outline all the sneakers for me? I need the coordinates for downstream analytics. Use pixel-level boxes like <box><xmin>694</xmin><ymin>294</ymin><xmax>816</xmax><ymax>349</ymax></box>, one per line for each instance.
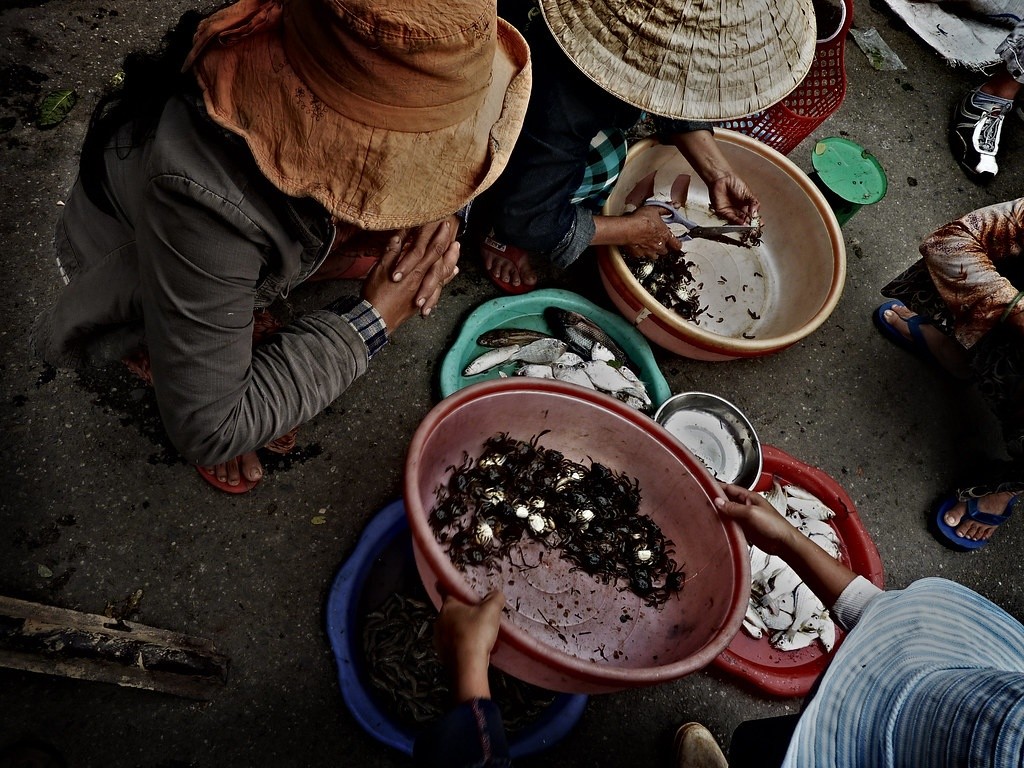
<box><xmin>949</xmin><ymin>84</ymin><xmax>1012</xmax><ymax>184</ymax></box>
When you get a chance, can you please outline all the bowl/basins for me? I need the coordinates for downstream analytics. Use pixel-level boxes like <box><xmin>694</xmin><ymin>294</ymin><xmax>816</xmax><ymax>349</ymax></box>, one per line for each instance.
<box><xmin>401</xmin><ymin>376</ymin><xmax>752</xmax><ymax>695</ymax></box>
<box><xmin>440</xmin><ymin>287</ymin><xmax>672</xmax><ymax>421</ymax></box>
<box><xmin>712</xmin><ymin>444</ymin><xmax>885</xmax><ymax>695</ymax></box>
<box><xmin>328</xmin><ymin>501</ymin><xmax>591</xmax><ymax>761</ymax></box>
<box><xmin>603</xmin><ymin>126</ymin><xmax>848</xmax><ymax>363</ymax></box>
<box><xmin>655</xmin><ymin>391</ymin><xmax>763</xmax><ymax>492</ymax></box>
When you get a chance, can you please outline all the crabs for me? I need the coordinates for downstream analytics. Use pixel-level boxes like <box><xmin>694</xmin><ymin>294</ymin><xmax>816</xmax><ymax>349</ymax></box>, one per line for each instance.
<box><xmin>426</xmin><ymin>424</ymin><xmax>689</xmax><ymax>612</ymax></box>
<box><xmin>737</xmin><ymin>214</ymin><xmax>764</xmax><ymax>247</ymax></box>
<box><xmin>613</xmin><ymin>241</ymin><xmax>710</xmax><ymax>326</ymax></box>
<box><xmin>367</xmin><ymin>571</ymin><xmax>563</xmax><ymax>746</ymax></box>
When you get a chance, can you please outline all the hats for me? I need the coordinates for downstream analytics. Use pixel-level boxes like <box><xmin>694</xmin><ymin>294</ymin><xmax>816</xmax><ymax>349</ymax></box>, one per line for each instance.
<box><xmin>777</xmin><ymin>575</ymin><xmax>1024</xmax><ymax>767</ymax></box>
<box><xmin>182</xmin><ymin>1</ymin><xmax>533</xmax><ymax>232</ymax></box>
<box><xmin>540</xmin><ymin>1</ymin><xmax>818</xmax><ymax>123</ymax></box>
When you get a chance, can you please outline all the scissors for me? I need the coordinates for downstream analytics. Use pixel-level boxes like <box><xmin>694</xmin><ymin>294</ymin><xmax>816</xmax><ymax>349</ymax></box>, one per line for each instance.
<box><xmin>644</xmin><ymin>200</ymin><xmax>753</xmax><ymax>249</ymax></box>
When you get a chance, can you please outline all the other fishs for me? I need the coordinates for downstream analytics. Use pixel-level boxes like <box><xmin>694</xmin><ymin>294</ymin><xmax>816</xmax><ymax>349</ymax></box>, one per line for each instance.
<box><xmin>459</xmin><ymin>306</ymin><xmax>655</xmax><ymax>414</ymax></box>
<box><xmin>741</xmin><ymin>473</ymin><xmax>843</xmax><ymax>654</ymax></box>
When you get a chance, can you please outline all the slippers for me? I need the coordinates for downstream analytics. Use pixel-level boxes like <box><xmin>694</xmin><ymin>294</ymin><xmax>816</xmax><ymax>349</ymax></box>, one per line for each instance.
<box><xmin>935</xmin><ymin>493</ymin><xmax>1019</xmax><ymax>550</ymax></box>
<box><xmin>875</xmin><ymin>299</ymin><xmax>957</xmax><ymax>373</ymax></box>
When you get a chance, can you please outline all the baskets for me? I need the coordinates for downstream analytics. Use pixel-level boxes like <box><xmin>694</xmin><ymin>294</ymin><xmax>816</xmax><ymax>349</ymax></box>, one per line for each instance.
<box><xmin>714</xmin><ymin>0</ymin><xmax>854</xmax><ymax>157</ymax></box>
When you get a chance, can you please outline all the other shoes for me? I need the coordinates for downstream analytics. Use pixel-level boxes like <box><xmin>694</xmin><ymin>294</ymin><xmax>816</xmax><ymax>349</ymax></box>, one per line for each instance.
<box><xmin>671</xmin><ymin>722</ymin><xmax>729</xmax><ymax>768</ymax></box>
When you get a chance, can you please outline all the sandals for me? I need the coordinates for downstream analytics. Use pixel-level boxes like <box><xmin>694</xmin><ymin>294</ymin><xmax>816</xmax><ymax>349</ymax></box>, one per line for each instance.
<box><xmin>197</xmin><ymin>466</ymin><xmax>260</xmax><ymax>495</ymax></box>
<box><xmin>479</xmin><ymin>229</ymin><xmax>537</xmax><ymax>294</ymax></box>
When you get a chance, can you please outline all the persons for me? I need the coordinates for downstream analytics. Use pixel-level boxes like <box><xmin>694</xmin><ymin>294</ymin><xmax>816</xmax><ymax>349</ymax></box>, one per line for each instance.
<box><xmin>435</xmin><ymin>485</ymin><xmax>1024</xmax><ymax>768</ymax></box>
<box><xmin>480</xmin><ymin>0</ymin><xmax>816</xmax><ymax>296</ymax></box>
<box><xmin>875</xmin><ymin>197</ymin><xmax>1024</xmax><ymax>551</ymax></box>
<box><xmin>909</xmin><ymin>0</ymin><xmax>1024</xmax><ymax>187</ymax></box>
<box><xmin>53</xmin><ymin>1</ymin><xmax>533</xmax><ymax>493</ymax></box>
